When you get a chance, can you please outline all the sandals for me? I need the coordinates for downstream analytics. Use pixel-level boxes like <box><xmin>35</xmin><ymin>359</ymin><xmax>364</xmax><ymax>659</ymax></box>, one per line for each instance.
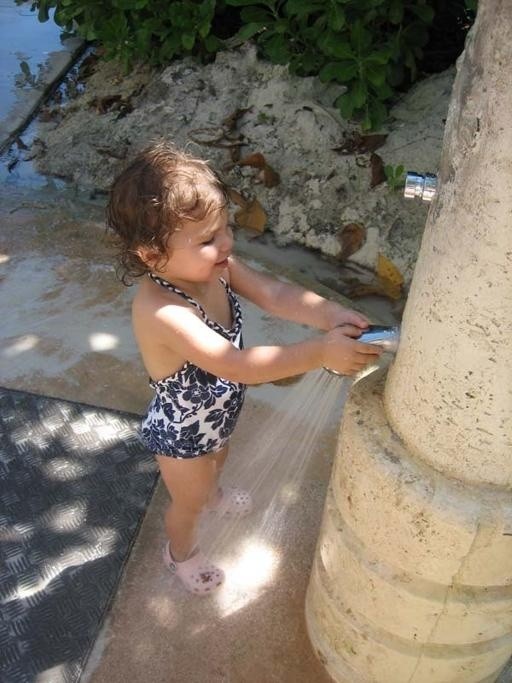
<box><xmin>162</xmin><ymin>537</ymin><xmax>226</xmax><ymax>596</ymax></box>
<box><xmin>200</xmin><ymin>483</ymin><xmax>255</xmax><ymax>519</ymax></box>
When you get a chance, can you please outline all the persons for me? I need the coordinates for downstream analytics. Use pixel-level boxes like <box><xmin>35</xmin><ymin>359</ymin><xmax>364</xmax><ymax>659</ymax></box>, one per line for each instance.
<box><xmin>110</xmin><ymin>144</ymin><xmax>383</xmax><ymax>595</ymax></box>
